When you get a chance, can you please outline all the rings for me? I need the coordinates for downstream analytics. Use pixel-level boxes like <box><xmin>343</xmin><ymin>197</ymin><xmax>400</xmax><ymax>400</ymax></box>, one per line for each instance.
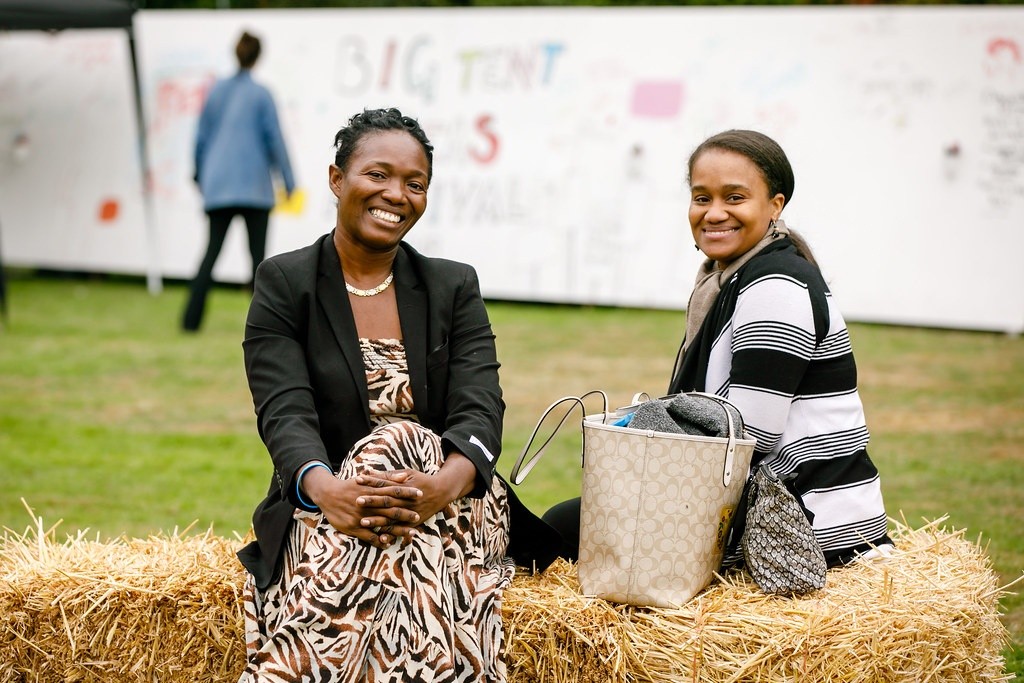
<box><xmin>372</xmin><ymin>525</ymin><xmax>382</xmax><ymax>533</ymax></box>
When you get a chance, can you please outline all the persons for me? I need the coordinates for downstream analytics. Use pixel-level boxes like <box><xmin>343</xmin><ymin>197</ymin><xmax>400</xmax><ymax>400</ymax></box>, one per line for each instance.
<box><xmin>543</xmin><ymin>129</ymin><xmax>895</xmax><ymax>577</ymax></box>
<box><xmin>235</xmin><ymin>106</ymin><xmax>515</xmax><ymax>683</ymax></box>
<box><xmin>183</xmin><ymin>31</ymin><xmax>297</xmax><ymax>331</ymax></box>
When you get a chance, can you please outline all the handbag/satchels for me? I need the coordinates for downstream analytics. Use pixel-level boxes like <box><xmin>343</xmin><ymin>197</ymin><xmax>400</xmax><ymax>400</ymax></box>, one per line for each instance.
<box><xmin>510</xmin><ymin>391</ymin><xmax>756</xmax><ymax>611</ymax></box>
<box><xmin>728</xmin><ymin>463</ymin><xmax>828</xmax><ymax>596</ymax></box>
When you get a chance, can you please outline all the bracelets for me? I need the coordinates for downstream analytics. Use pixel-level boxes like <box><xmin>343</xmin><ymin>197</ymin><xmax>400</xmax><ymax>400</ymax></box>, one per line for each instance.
<box><xmin>297</xmin><ymin>463</ymin><xmax>332</xmax><ymax>509</ymax></box>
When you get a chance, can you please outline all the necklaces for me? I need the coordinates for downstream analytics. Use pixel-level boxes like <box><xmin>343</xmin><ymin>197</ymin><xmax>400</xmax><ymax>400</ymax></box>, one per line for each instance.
<box><xmin>345</xmin><ymin>269</ymin><xmax>394</xmax><ymax>297</ymax></box>
